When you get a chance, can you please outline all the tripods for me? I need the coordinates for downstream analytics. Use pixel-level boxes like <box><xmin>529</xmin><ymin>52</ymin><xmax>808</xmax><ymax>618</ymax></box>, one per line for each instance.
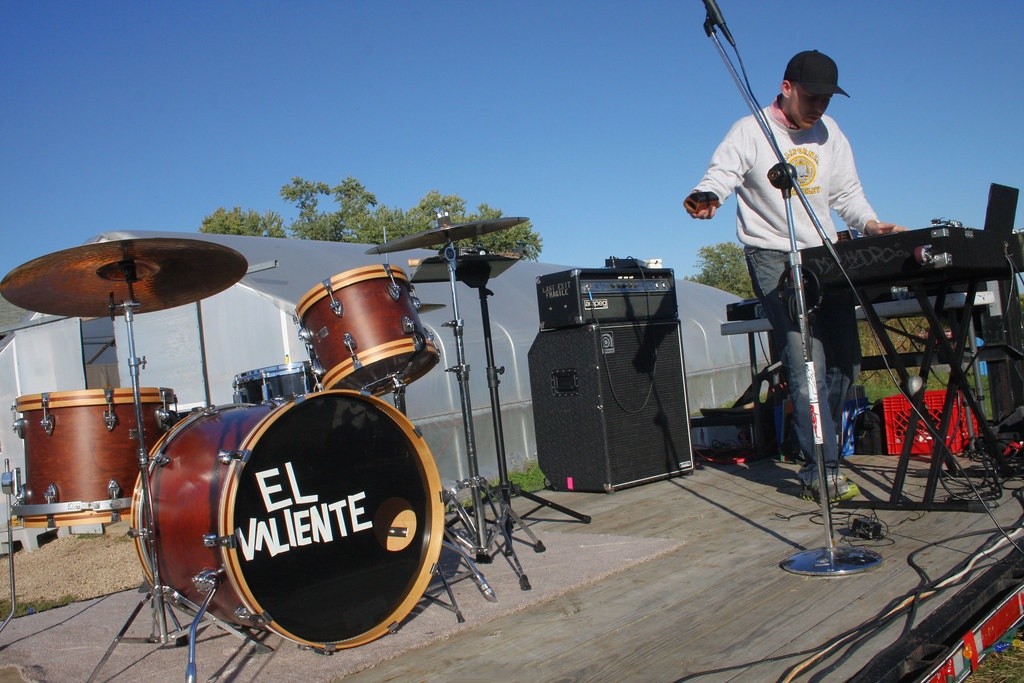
<box><xmin>83</xmin><ymin>264</ymin><xmax>275</xmax><ymax>683</ymax></box>
<box><xmin>436</xmin><ymin>237</ymin><xmax>592</xmax><ymax>589</ymax></box>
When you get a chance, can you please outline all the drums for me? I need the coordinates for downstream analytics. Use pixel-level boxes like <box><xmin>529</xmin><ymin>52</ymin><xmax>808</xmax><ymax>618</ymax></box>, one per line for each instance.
<box><xmin>231</xmin><ymin>359</ymin><xmax>318</xmax><ymax>405</ymax></box>
<box><xmin>11</xmin><ymin>384</ymin><xmax>176</xmax><ymax>527</ymax></box>
<box><xmin>130</xmin><ymin>389</ymin><xmax>445</xmax><ymax>654</ymax></box>
<box><xmin>290</xmin><ymin>262</ymin><xmax>441</xmax><ymax>401</ymax></box>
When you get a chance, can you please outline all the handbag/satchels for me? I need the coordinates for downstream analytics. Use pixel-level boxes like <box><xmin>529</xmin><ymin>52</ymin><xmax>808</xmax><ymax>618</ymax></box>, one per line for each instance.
<box><xmin>852</xmin><ymin>408</ymin><xmax>883</xmax><ymax>455</ymax></box>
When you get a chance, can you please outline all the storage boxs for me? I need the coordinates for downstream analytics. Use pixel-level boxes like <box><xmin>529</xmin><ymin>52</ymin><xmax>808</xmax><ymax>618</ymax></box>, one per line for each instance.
<box><xmin>691</xmin><ymin>415</ymin><xmax>753</xmax><ymax>450</ymax></box>
<box><xmin>842</xmin><ymin>397</ymin><xmax>871</xmax><ymax>456</ymax></box>
<box><xmin>882</xmin><ymin>388</ymin><xmax>980</xmax><ymax>456</ymax></box>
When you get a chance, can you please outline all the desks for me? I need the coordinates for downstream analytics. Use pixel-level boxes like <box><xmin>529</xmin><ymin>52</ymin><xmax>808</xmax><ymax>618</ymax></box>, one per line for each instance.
<box><xmin>720</xmin><ymin>289</ymin><xmax>995</xmax><ymax>463</ymax></box>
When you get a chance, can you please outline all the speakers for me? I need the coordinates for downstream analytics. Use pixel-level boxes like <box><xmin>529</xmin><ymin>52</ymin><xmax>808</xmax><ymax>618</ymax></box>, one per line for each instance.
<box><xmin>528</xmin><ymin>317</ymin><xmax>694</xmax><ymax>495</ymax></box>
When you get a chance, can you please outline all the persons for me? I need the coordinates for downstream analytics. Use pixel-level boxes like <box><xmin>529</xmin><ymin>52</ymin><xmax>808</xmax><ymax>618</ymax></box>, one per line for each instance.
<box><xmin>683</xmin><ymin>49</ymin><xmax>906</xmax><ymax>504</ymax></box>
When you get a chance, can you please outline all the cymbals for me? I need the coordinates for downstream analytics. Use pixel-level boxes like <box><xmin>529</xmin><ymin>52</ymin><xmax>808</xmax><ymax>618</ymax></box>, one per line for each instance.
<box><xmin>1</xmin><ymin>237</ymin><xmax>247</xmax><ymax>318</ymax></box>
<box><xmin>417</xmin><ymin>303</ymin><xmax>447</xmax><ymax>314</ymax></box>
<box><xmin>364</xmin><ymin>217</ymin><xmax>532</xmax><ymax>257</ymax></box>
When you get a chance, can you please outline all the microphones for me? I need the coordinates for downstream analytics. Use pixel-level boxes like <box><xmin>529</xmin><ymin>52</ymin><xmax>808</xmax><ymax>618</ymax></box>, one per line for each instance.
<box><xmin>702</xmin><ymin>0</ymin><xmax>736</xmax><ymax>46</ymax></box>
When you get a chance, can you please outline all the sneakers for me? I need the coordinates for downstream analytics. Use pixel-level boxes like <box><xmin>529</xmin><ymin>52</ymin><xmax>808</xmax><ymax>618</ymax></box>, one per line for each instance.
<box><xmin>798</xmin><ymin>463</ymin><xmax>847</xmax><ymax>486</ymax></box>
<box><xmin>800</xmin><ymin>467</ymin><xmax>860</xmax><ymax>502</ymax></box>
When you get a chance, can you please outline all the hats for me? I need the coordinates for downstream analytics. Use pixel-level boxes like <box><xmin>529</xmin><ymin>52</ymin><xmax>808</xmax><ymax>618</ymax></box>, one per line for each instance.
<box><xmin>784</xmin><ymin>50</ymin><xmax>851</xmax><ymax>98</ymax></box>
